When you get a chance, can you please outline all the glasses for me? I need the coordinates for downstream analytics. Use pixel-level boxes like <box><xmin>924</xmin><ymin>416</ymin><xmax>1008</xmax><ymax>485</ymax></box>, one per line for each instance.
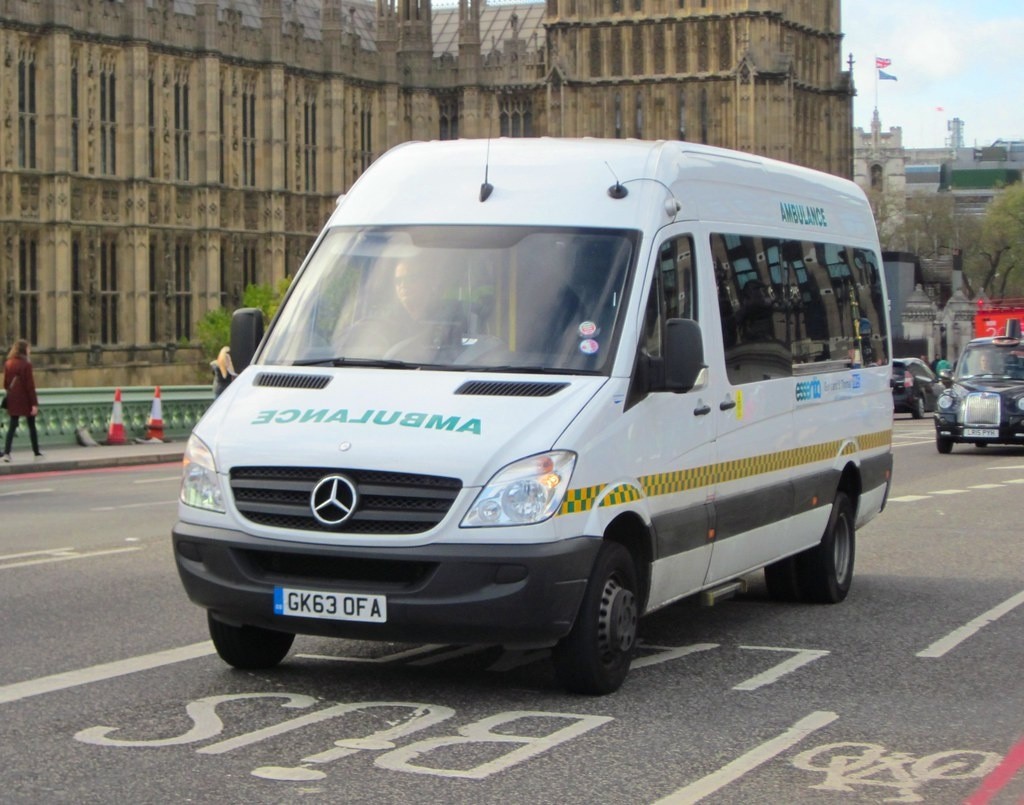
<box><xmin>392</xmin><ymin>273</ymin><xmax>419</xmax><ymax>285</ymax></box>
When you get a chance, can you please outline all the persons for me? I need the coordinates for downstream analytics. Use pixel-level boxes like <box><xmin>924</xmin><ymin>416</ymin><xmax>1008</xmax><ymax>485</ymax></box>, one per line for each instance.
<box><xmin>931</xmin><ymin>354</ymin><xmax>941</xmax><ymax>371</ymax></box>
<box><xmin>209</xmin><ymin>346</ymin><xmax>231</xmax><ymax>401</ymax></box>
<box><xmin>390</xmin><ymin>255</ymin><xmax>462</xmax><ymax>361</ymax></box>
<box><xmin>735</xmin><ymin>279</ymin><xmax>777</xmax><ymax>347</ymax></box>
<box><xmin>980</xmin><ymin>356</ymin><xmax>993</xmax><ymax>374</ymax></box>
<box><xmin>3</xmin><ymin>340</ymin><xmax>43</xmax><ymax>462</ymax></box>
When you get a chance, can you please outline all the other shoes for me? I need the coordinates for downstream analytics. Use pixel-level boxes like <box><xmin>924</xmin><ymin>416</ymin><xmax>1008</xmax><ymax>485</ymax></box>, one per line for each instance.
<box><xmin>34</xmin><ymin>453</ymin><xmax>44</xmax><ymax>459</ymax></box>
<box><xmin>3</xmin><ymin>454</ymin><xmax>12</xmax><ymax>462</ymax></box>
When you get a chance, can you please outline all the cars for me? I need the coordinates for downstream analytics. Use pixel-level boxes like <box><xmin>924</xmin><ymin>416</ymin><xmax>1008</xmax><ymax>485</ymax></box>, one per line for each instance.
<box><xmin>891</xmin><ymin>357</ymin><xmax>948</xmax><ymax>420</ymax></box>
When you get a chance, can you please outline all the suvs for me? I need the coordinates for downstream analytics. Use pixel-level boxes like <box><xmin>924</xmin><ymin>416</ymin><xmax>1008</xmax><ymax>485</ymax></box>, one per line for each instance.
<box><xmin>932</xmin><ymin>335</ymin><xmax>1024</xmax><ymax>453</ymax></box>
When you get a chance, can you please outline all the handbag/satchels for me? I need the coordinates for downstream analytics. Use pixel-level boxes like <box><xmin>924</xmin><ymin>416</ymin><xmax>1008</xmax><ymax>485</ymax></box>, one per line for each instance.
<box><xmin>0</xmin><ymin>396</ymin><xmax>8</xmax><ymax>409</ymax></box>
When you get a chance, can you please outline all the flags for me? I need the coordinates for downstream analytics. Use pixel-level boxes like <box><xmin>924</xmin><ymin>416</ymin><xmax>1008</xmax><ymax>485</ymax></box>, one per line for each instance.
<box><xmin>876</xmin><ymin>57</ymin><xmax>891</xmax><ymax>68</ymax></box>
<box><xmin>880</xmin><ymin>70</ymin><xmax>897</xmax><ymax>81</ymax></box>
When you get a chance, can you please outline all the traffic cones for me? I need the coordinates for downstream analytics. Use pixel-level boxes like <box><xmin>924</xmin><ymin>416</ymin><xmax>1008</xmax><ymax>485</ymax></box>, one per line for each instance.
<box><xmin>134</xmin><ymin>384</ymin><xmax>173</xmax><ymax>444</ymax></box>
<box><xmin>102</xmin><ymin>388</ymin><xmax>131</xmax><ymax>446</ymax></box>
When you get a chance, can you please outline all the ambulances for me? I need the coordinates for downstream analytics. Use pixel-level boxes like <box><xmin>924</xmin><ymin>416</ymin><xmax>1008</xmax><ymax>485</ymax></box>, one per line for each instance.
<box><xmin>168</xmin><ymin>135</ymin><xmax>895</xmax><ymax>697</ymax></box>
